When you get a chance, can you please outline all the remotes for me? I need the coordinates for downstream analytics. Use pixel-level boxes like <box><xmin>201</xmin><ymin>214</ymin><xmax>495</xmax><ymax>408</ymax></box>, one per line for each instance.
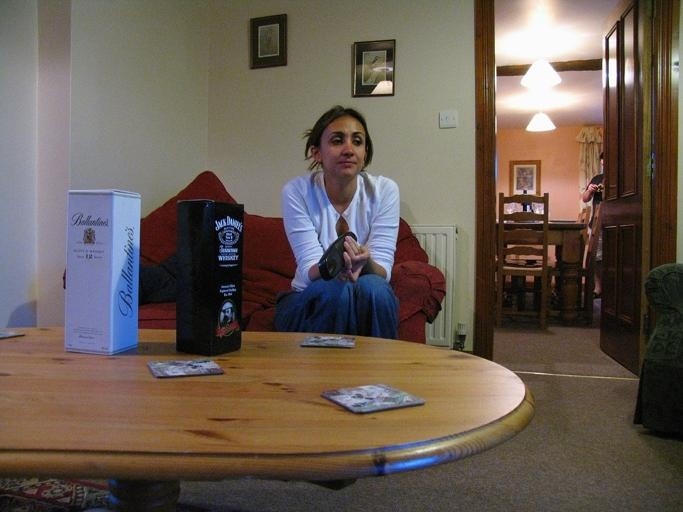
<box><xmin>319</xmin><ymin>230</ymin><xmax>358</xmax><ymax>281</ymax></box>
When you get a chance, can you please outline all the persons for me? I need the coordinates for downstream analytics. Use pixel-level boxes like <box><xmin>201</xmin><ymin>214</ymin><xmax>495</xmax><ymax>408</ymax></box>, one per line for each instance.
<box><xmin>582</xmin><ymin>152</ymin><xmax>605</xmax><ymax>298</ymax></box>
<box><xmin>222</xmin><ymin>301</ymin><xmax>234</xmax><ymax>324</ymax></box>
<box><xmin>274</xmin><ymin>105</ymin><xmax>400</xmax><ymax>340</ymax></box>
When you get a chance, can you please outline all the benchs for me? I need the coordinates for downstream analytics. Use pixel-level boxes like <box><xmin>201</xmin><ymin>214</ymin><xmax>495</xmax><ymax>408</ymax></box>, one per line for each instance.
<box><xmin>63</xmin><ymin>212</ymin><xmax>449</xmax><ymax>345</ymax></box>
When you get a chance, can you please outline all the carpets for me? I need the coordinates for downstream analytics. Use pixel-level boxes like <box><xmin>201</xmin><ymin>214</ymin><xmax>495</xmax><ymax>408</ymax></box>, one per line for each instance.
<box><xmin>0</xmin><ymin>473</ymin><xmax>114</xmax><ymax>511</ymax></box>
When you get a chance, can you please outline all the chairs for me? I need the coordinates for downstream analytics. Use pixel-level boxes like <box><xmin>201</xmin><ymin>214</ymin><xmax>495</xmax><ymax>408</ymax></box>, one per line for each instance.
<box><xmin>575</xmin><ymin>205</ymin><xmax>592</xmax><ymax>265</ymax></box>
<box><xmin>545</xmin><ymin>200</ymin><xmax>604</xmax><ymax>310</ymax></box>
<box><xmin>497</xmin><ymin>191</ymin><xmax>552</xmax><ymax>331</ymax></box>
<box><xmin>627</xmin><ymin>263</ymin><xmax>682</xmax><ymax>444</ymax></box>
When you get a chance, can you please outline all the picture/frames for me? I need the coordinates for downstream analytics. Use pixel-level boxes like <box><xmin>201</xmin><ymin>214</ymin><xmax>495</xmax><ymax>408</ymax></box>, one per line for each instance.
<box><xmin>350</xmin><ymin>38</ymin><xmax>396</xmax><ymax>98</ymax></box>
<box><xmin>250</xmin><ymin>13</ymin><xmax>288</xmax><ymax>70</ymax></box>
<box><xmin>509</xmin><ymin>159</ymin><xmax>542</xmax><ymax>196</ymax></box>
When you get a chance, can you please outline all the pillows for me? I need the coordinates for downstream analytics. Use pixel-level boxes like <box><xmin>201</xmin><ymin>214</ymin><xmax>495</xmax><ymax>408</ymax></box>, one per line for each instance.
<box><xmin>139</xmin><ymin>168</ymin><xmax>245</xmax><ymax>264</ymax></box>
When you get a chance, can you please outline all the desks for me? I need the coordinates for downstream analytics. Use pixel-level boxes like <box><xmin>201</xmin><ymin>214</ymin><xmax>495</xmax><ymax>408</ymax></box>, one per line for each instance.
<box><xmin>493</xmin><ymin>220</ymin><xmax>588</xmax><ymax>327</ymax></box>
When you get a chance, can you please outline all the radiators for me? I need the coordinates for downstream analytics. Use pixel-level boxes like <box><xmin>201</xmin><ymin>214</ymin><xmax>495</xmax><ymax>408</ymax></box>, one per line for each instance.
<box><xmin>405</xmin><ymin>225</ymin><xmax>458</xmax><ymax>347</ymax></box>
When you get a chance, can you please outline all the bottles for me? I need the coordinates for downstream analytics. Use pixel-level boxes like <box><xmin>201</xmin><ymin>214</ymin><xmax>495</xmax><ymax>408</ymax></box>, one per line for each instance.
<box><xmin>523</xmin><ymin>190</ymin><xmax>530</xmax><ymax>212</ymax></box>
